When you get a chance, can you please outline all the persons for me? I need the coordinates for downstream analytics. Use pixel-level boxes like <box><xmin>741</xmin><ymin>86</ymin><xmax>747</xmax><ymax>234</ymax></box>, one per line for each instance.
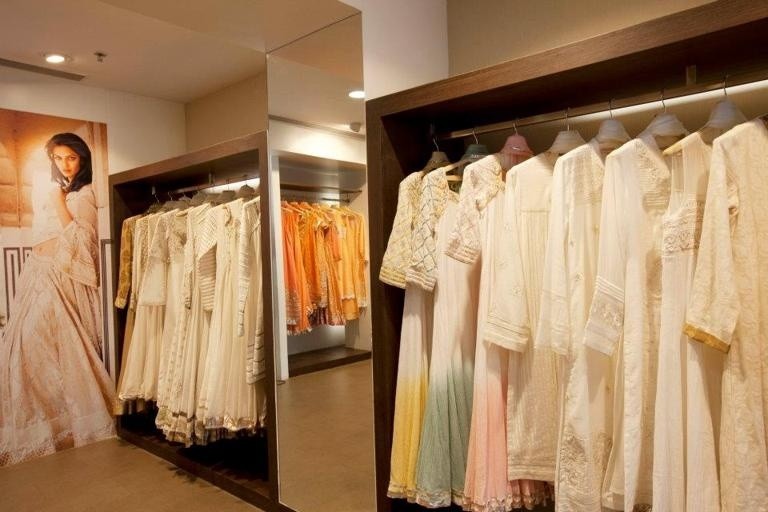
<box><xmin>0</xmin><ymin>131</ymin><xmax>119</xmax><ymax>468</ymax></box>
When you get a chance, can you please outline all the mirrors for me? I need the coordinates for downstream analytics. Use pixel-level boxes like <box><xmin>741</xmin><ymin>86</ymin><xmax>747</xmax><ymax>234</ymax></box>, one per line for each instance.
<box><xmin>265</xmin><ymin>11</ymin><xmax>377</xmax><ymax>512</ymax></box>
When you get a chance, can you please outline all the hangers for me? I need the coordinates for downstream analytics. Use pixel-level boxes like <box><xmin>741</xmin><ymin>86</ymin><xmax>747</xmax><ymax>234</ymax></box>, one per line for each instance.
<box><xmin>155</xmin><ymin>174</ymin><xmax>248</xmax><ymax>217</ymax></box>
<box><xmin>405</xmin><ymin>72</ymin><xmax>768</xmax><ymax>182</ymax></box>
<box><xmin>283</xmin><ymin>190</ymin><xmax>351</xmax><ymax>208</ymax></box>
